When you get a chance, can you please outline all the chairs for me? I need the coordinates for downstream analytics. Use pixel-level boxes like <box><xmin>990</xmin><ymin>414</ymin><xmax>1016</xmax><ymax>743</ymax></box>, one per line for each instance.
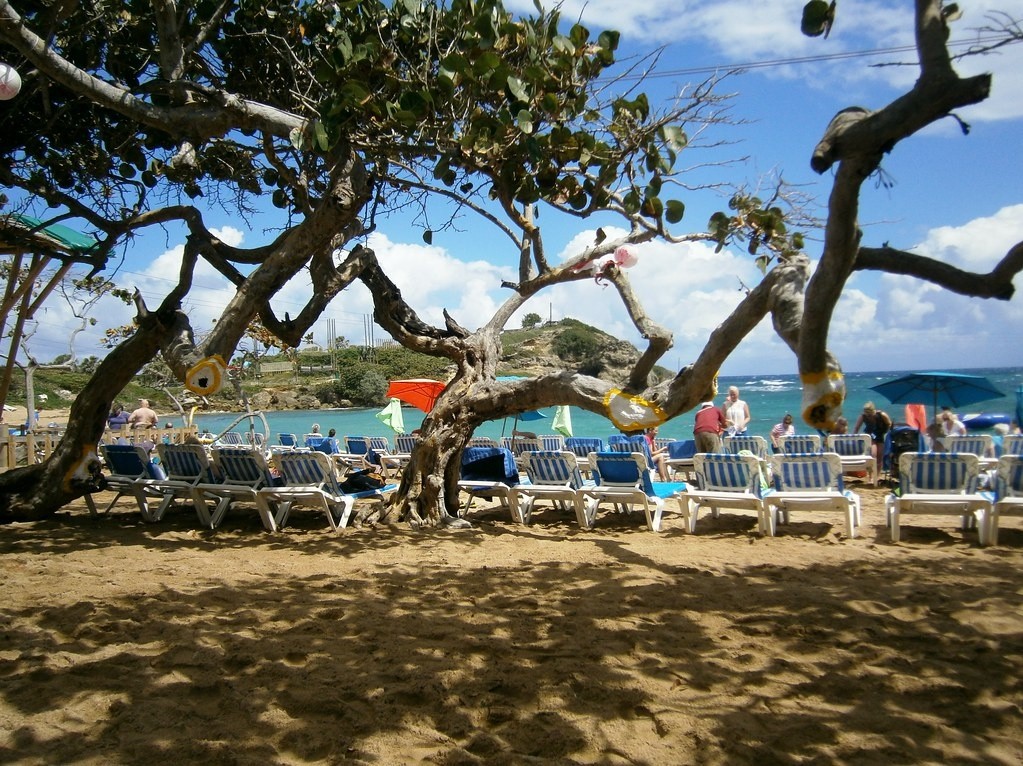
<box><xmin>0</xmin><ymin>423</ymin><xmax>1022</xmax><ymax>531</ymax></box>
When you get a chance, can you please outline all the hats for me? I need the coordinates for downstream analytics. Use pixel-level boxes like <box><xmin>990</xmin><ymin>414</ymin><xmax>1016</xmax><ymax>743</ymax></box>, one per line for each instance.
<box><xmin>702</xmin><ymin>401</ymin><xmax>714</xmax><ymax>408</ymax></box>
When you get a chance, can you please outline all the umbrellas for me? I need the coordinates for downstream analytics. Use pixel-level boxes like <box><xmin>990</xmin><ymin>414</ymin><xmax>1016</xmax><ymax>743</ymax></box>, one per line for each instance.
<box><xmin>868</xmin><ymin>372</ymin><xmax>1005</xmax><ymax>424</ymax></box>
<box><xmin>384</xmin><ymin>378</ymin><xmax>445</xmax><ymax>413</ymax></box>
<box><xmin>496</xmin><ymin>376</ymin><xmax>548</xmax><ymax>438</ymax></box>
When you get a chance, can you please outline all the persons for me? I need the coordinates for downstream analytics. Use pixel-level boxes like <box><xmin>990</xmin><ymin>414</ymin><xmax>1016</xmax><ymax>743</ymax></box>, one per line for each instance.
<box><xmin>308</xmin><ymin>424</ymin><xmax>339</xmax><ymax>452</ymax></box>
<box><xmin>512</xmin><ymin>430</ymin><xmax>537</xmax><ymax>451</ymax></box>
<box><xmin>721</xmin><ymin>385</ymin><xmax>751</xmax><ymax>436</ymax></box>
<box><xmin>25</xmin><ymin>406</ymin><xmax>42</xmax><ymax>428</ymax></box>
<box><xmin>105</xmin><ymin>399</ymin><xmax>175</xmax><ymax>443</ymax></box>
<box><xmin>930</xmin><ymin>406</ymin><xmax>966</xmax><ymax>435</ymax></box>
<box><xmin>852</xmin><ymin>401</ymin><xmax>891</xmax><ymax>484</ymax></box>
<box><xmin>770</xmin><ymin>415</ymin><xmax>795</xmax><ymax>453</ymax></box>
<box><xmin>202</xmin><ymin>429</ymin><xmax>226</xmax><ymax>443</ymax></box>
<box><xmin>694</xmin><ymin>400</ymin><xmax>727</xmax><ymax>453</ymax></box>
<box><xmin>645</xmin><ymin>426</ymin><xmax>671</xmax><ymax>482</ymax></box>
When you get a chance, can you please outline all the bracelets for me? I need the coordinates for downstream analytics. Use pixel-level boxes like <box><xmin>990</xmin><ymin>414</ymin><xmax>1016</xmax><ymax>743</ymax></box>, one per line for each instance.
<box><xmin>517</xmin><ymin>433</ymin><xmax>518</xmax><ymax>435</ymax></box>
